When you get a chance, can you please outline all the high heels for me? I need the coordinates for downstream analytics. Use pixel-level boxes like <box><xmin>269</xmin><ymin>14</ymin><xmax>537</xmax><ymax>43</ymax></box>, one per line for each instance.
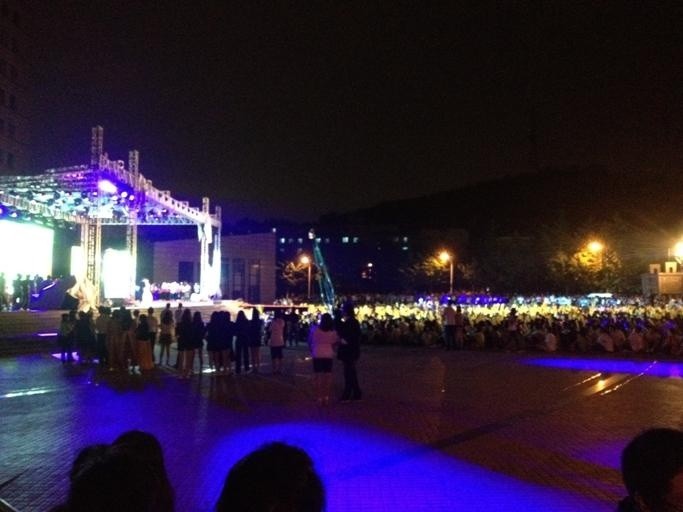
<box><xmin>315</xmin><ymin>396</ymin><xmax>329</xmax><ymax>406</ymax></box>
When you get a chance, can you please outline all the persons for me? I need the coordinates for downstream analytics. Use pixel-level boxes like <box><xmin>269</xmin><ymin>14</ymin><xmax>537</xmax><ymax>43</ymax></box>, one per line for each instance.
<box><xmin>214</xmin><ymin>442</ymin><xmax>326</xmax><ymax>512</ymax></box>
<box><xmin>616</xmin><ymin>426</ymin><xmax>683</xmax><ymax>512</ymax></box>
<box><xmin>66</xmin><ymin>426</ymin><xmax>176</xmax><ymax>511</ymax></box>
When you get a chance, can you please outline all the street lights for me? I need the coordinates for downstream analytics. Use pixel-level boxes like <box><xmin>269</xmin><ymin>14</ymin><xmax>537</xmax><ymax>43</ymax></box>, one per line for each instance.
<box><xmin>299</xmin><ymin>255</ymin><xmax>312</xmax><ymax>298</ymax></box>
<box><xmin>439</xmin><ymin>253</ymin><xmax>454</xmax><ymax>294</ymax></box>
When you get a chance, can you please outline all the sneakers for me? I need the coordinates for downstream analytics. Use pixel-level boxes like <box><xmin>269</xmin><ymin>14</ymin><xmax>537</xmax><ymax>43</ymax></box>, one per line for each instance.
<box><xmin>339</xmin><ymin>395</ymin><xmax>363</xmax><ymax>404</ymax></box>
